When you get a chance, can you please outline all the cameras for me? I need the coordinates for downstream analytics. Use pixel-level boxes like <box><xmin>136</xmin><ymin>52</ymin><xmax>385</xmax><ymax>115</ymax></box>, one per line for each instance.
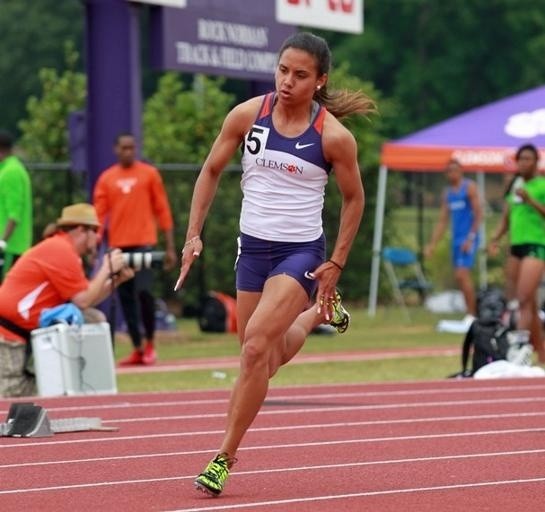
<box><xmin>104</xmin><ymin>249</ymin><xmax>166</xmax><ymax>271</ymax></box>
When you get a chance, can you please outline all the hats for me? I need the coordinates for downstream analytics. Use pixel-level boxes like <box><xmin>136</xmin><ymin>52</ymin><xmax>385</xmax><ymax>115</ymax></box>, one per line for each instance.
<box><xmin>55</xmin><ymin>202</ymin><xmax>102</xmax><ymax>229</ymax></box>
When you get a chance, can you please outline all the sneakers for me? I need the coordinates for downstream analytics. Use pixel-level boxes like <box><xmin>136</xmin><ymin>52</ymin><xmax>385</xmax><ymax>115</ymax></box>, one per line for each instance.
<box><xmin>193</xmin><ymin>451</ymin><xmax>238</xmax><ymax>498</ymax></box>
<box><xmin>117</xmin><ymin>349</ymin><xmax>142</xmax><ymax>368</ymax></box>
<box><xmin>141</xmin><ymin>342</ymin><xmax>156</xmax><ymax>366</ymax></box>
<box><xmin>318</xmin><ymin>287</ymin><xmax>351</xmax><ymax>334</ymax></box>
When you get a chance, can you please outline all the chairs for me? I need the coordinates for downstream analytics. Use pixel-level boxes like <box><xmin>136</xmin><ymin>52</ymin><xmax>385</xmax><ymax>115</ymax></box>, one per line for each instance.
<box><xmin>381</xmin><ymin>246</ymin><xmax>437</xmax><ymax>325</ymax></box>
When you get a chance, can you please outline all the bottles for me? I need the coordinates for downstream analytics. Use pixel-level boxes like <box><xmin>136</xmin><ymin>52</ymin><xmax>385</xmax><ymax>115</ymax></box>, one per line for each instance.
<box><xmin>511</xmin><ymin>175</ymin><xmax>524</xmax><ymax>204</ymax></box>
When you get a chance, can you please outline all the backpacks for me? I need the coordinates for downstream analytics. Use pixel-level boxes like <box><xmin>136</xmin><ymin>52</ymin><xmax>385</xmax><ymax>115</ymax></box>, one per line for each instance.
<box><xmin>449</xmin><ymin>320</ymin><xmax>508</xmax><ymax>382</ymax></box>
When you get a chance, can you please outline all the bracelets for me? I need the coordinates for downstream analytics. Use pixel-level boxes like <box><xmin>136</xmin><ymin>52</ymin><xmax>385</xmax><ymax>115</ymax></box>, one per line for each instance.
<box><xmin>185</xmin><ymin>235</ymin><xmax>200</xmax><ymax>245</ymax></box>
<box><xmin>329</xmin><ymin>260</ymin><xmax>343</xmax><ymax>271</ymax></box>
<box><xmin>467</xmin><ymin>232</ymin><xmax>476</xmax><ymax>241</ymax></box>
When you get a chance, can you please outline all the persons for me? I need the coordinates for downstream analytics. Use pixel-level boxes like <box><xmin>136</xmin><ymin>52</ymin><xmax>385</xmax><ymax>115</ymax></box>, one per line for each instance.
<box><xmin>0</xmin><ymin>204</ymin><xmax>135</xmax><ymax>398</ymax></box>
<box><xmin>425</xmin><ymin>158</ymin><xmax>484</xmax><ymax>322</ymax></box>
<box><xmin>488</xmin><ymin>144</ymin><xmax>545</xmax><ymax>365</ymax></box>
<box><xmin>92</xmin><ymin>135</ymin><xmax>178</xmax><ymax>367</ymax></box>
<box><xmin>0</xmin><ymin>129</ymin><xmax>33</xmax><ymax>289</ymax></box>
<box><xmin>173</xmin><ymin>32</ymin><xmax>381</xmax><ymax>496</ymax></box>
